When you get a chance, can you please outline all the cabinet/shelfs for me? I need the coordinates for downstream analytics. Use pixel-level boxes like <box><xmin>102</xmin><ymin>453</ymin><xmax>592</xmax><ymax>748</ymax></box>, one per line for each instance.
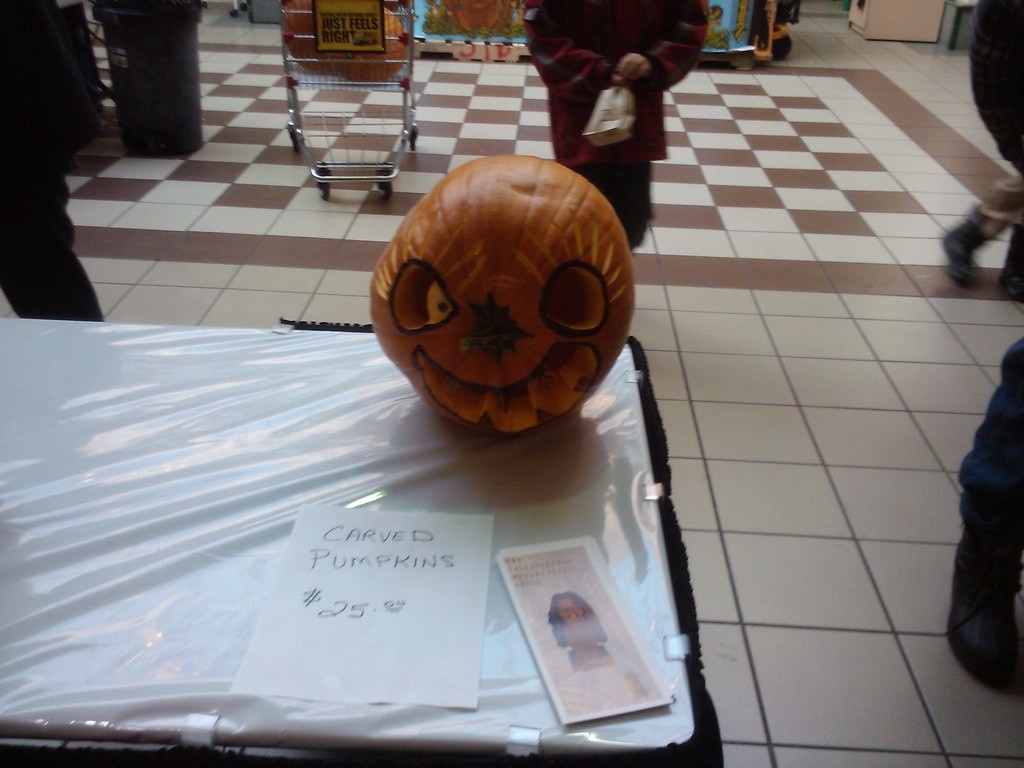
<box><xmin>848</xmin><ymin>0</ymin><xmax>946</xmax><ymax>42</ymax></box>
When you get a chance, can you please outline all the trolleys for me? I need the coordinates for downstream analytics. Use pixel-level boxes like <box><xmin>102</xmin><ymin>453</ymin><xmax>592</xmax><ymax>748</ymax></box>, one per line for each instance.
<box><xmin>281</xmin><ymin>0</ymin><xmax>418</xmax><ymax>201</ymax></box>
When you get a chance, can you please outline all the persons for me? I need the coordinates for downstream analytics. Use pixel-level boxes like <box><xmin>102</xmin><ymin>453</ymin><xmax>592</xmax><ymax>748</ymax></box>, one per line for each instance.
<box><xmin>941</xmin><ymin>0</ymin><xmax>1024</xmax><ymax>301</ymax></box>
<box><xmin>518</xmin><ymin>0</ymin><xmax>711</xmax><ymax>250</ymax></box>
<box><xmin>1</xmin><ymin>0</ymin><xmax>104</xmax><ymax>322</ymax></box>
<box><xmin>947</xmin><ymin>339</ymin><xmax>1024</xmax><ymax>696</ymax></box>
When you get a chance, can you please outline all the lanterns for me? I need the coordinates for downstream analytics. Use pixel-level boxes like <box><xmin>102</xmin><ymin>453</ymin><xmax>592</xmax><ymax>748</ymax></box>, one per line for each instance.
<box><xmin>369</xmin><ymin>154</ymin><xmax>635</xmax><ymax>439</ymax></box>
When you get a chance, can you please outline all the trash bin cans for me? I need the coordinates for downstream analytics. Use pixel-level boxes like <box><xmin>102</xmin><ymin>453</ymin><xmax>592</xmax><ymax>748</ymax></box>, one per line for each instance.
<box><xmin>91</xmin><ymin>0</ymin><xmax>203</xmax><ymax>156</ymax></box>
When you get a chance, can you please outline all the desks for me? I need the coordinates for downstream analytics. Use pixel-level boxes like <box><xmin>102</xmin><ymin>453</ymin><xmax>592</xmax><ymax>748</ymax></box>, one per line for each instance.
<box><xmin>0</xmin><ymin>316</ymin><xmax>724</xmax><ymax>768</ymax></box>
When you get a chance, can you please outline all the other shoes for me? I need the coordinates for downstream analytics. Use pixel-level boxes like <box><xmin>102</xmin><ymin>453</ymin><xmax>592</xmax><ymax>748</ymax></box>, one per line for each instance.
<box><xmin>944</xmin><ymin>561</ymin><xmax>1019</xmax><ymax>687</ymax></box>
<box><xmin>999</xmin><ymin>266</ymin><xmax>1024</xmax><ymax>301</ymax></box>
<box><xmin>944</xmin><ymin>220</ymin><xmax>986</xmax><ymax>288</ymax></box>
